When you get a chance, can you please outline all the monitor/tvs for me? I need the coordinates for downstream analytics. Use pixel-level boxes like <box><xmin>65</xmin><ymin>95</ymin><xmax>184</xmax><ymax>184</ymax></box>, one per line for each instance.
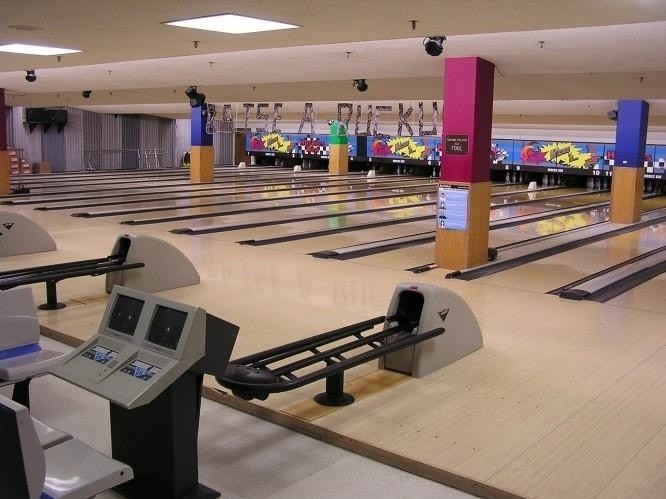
<box><xmin>104</xmin><ymin>294</ymin><xmax>145</xmax><ymax>336</ymax></box>
<box><xmin>144</xmin><ymin>304</ymin><xmax>188</xmax><ymax>352</ymax></box>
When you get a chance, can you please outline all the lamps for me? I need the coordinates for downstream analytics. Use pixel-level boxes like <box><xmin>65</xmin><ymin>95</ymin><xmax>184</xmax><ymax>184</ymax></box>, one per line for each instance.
<box><xmin>24</xmin><ymin>69</ymin><xmax>37</xmax><ymax>83</ymax></box>
<box><xmin>423</xmin><ymin>36</ymin><xmax>447</xmax><ymax>56</ymax></box>
<box><xmin>185</xmin><ymin>85</ymin><xmax>206</xmax><ymax>108</ymax></box>
<box><xmin>353</xmin><ymin>79</ymin><xmax>368</xmax><ymax>91</ymax></box>
<box><xmin>82</xmin><ymin>89</ymin><xmax>92</xmax><ymax>98</ymax></box>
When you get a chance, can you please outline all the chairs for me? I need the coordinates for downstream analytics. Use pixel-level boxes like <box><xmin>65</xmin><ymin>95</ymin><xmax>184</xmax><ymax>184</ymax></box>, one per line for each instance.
<box><xmin>0</xmin><ymin>287</ymin><xmax>134</xmax><ymax>499</ymax></box>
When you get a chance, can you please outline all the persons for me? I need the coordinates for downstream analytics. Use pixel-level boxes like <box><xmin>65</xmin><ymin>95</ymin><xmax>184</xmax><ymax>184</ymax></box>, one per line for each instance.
<box><xmin>439</xmin><ymin>193</ymin><xmax>446</xmax><ymax>201</ymax></box>
<box><xmin>438</xmin><ymin>202</ymin><xmax>445</xmax><ymax>209</ymax></box>
<box><xmin>438</xmin><ymin>211</ymin><xmax>446</xmax><ymax>219</ymax></box>
<box><xmin>438</xmin><ymin>220</ymin><xmax>445</xmax><ymax>228</ymax></box>
